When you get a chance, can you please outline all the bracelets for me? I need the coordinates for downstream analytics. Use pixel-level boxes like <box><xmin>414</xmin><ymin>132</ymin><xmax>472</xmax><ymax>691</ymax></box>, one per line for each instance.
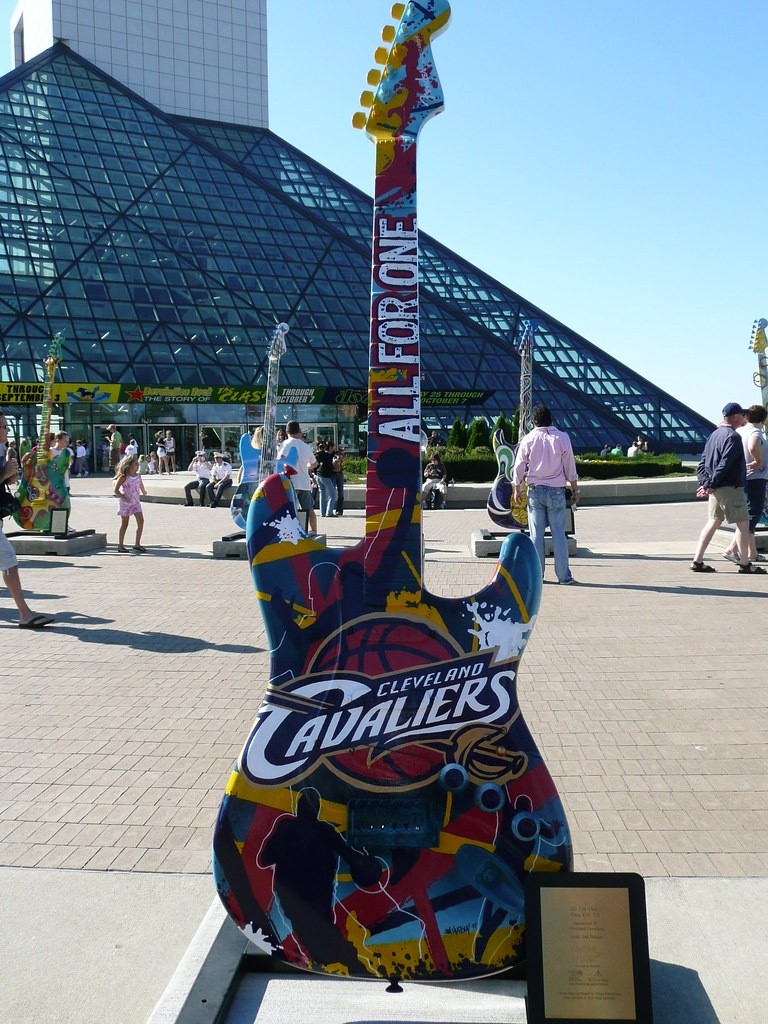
<box><xmin>572</xmin><ymin>488</ymin><xmax>580</xmax><ymax>493</ymax></box>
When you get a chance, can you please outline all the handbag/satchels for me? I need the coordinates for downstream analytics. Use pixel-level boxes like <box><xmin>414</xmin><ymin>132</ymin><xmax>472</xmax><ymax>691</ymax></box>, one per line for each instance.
<box><xmin>0</xmin><ymin>480</ymin><xmax>21</xmax><ymax>518</ymax></box>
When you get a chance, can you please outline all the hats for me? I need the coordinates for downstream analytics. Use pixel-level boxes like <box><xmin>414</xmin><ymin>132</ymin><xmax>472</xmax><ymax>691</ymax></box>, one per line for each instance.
<box><xmin>722</xmin><ymin>402</ymin><xmax>748</xmax><ymax>417</ymax></box>
<box><xmin>325</xmin><ymin>441</ymin><xmax>335</xmax><ymax>446</ymax></box>
<box><xmin>428</xmin><ymin>452</ymin><xmax>439</xmax><ymax>460</ymax></box>
<box><xmin>213</xmin><ymin>452</ymin><xmax>224</xmax><ymax>458</ymax></box>
<box><xmin>195</xmin><ymin>451</ymin><xmax>206</xmax><ymax>456</ymax></box>
<box><xmin>106</xmin><ymin>424</ymin><xmax>115</xmax><ymax>430</ymax></box>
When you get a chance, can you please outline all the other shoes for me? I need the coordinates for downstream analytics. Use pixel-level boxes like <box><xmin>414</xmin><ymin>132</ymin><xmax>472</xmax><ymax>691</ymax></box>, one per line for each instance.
<box><xmin>559</xmin><ymin>577</ymin><xmax>574</xmax><ymax>585</ymax></box>
<box><xmin>207</xmin><ymin>500</ymin><xmax>217</xmax><ymax>508</ymax></box>
<box><xmin>133</xmin><ymin>545</ymin><xmax>146</xmax><ymax>551</ymax></box>
<box><xmin>76</xmin><ymin>473</ymin><xmax>81</xmax><ymax>477</ymax></box>
<box><xmin>83</xmin><ymin>471</ymin><xmax>89</xmax><ymax>476</ymax></box>
<box><xmin>440</xmin><ymin>503</ymin><xmax>446</xmax><ymax>508</ymax></box>
<box><xmin>185</xmin><ymin>503</ymin><xmax>194</xmax><ymax>506</ymax></box>
<box><xmin>423</xmin><ymin>501</ymin><xmax>428</xmax><ymax>508</ymax></box>
<box><xmin>200</xmin><ymin>502</ymin><xmax>205</xmax><ymax>506</ymax></box>
<box><xmin>326</xmin><ymin>514</ymin><xmax>338</xmax><ymax>517</ymax></box>
<box><xmin>118</xmin><ymin>548</ymin><xmax>129</xmax><ymax>552</ymax></box>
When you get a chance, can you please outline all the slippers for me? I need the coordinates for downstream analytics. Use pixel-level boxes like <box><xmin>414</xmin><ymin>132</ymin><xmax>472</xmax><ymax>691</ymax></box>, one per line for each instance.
<box><xmin>19</xmin><ymin>616</ymin><xmax>53</xmax><ymax>628</ymax></box>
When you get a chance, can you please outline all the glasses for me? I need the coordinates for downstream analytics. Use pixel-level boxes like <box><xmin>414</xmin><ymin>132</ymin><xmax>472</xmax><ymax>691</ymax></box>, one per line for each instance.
<box><xmin>0</xmin><ymin>425</ymin><xmax>7</xmax><ymax>429</ymax></box>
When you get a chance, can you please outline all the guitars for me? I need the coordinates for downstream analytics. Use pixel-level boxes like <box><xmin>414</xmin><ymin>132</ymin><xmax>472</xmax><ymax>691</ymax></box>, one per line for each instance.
<box><xmin>214</xmin><ymin>0</ymin><xmax>576</xmax><ymax>995</ymax></box>
<box><xmin>230</xmin><ymin>321</ymin><xmax>301</xmax><ymax>534</ymax></box>
<box><xmin>486</xmin><ymin>319</ymin><xmax>575</xmax><ymax>531</ymax></box>
<box><xmin>748</xmin><ymin>316</ymin><xmax>768</xmax><ymax>527</ymax></box>
<box><xmin>10</xmin><ymin>326</ymin><xmax>73</xmax><ymax>531</ymax></box>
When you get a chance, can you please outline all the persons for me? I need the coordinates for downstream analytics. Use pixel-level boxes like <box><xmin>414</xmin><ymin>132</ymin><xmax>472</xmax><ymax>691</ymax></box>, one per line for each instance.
<box><xmin>428</xmin><ymin>431</ymin><xmax>446</xmax><ymax>447</ymax></box>
<box><xmin>512</xmin><ymin>405</ymin><xmax>581</xmax><ymax>584</ymax></box>
<box><xmin>689</xmin><ymin>402</ymin><xmax>767</xmax><ymax>574</ymax></box>
<box><xmin>296</xmin><ymin>440</ymin><xmax>344</xmax><ymax>518</ymax></box>
<box><xmin>105</xmin><ymin>425</ymin><xmax>175</xmax><ymax>476</ymax></box>
<box><xmin>33</xmin><ymin>431</ymin><xmax>75</xmax><ymax>531</ymax></box>
<box><xmin>76</xmin><ymin>438</ymin><xmax>90</xmax><ymax>478</ymax></box>
<box><xmin>201</xmin><ymin>430</ymin><xmax>210</xmax><ymax>462</ymax></box>
<box><xmin>114</xmin><ymin>454</ymin><xmax>147</xmax><ymax>553</ymax></box>
<box><xmin>276</xmin><ymin>420</ymin><xmax>318</xmax><ymax>536</ymax></box>
<box><xmin>627</xmin><ymin>435</ymin><xmax>648</xmax><ymax>458</ymax></box>
<box><xmin>0</xmin><ymin>409</ymin><xmax>54</xmax><ymax>629</ymax></box>
<box><xmin>183</xmin><ymin>451</ymin><xmax>212</xmax><ymax>506</ymax></box>
<box><xmin>225</xmin><ymin>436</ymin><xmax>237</xmax><ymax>463</ymax></box>
<box><xmin>207</xmin><ymin>452</ymin><xmax>232</xmax><ymax>508</ymax></box>
<box><xmin>422</xmin><ymin>454</ymin><xmax>447</xmax><ymax>509</ymax></box>
<box><xmin>722</xmin><ymin>405</ymin><xmax>768</xmax><ymax>564</ymax></box>
<box><xmin>251</xmin><ymin>426</ymin><xmax>285</xmax><ymax>453</ymax></box>
<box><xmin>601</xmin><ymin>444</ymin><xmax>623</xmax><ymax>457</ymax></box>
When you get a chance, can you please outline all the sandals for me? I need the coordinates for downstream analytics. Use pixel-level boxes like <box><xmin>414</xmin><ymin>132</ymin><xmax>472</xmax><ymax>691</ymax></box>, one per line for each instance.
<box><xmin>737</xmin><ymin>562</ymin><xmax>767</xmax><ymax>574</ymax></box>
<box><xmin>689</xmin><ymin>561</ymin><xmax>714</xmax><ymax>572</ymax></box>
<box><xmin>749</xmin><ymin>555</ymin><xmax>768</xmax><ymax>562</ymax></box>
<box><xmin>722</xmin><ymin>548</ymin><xmax>739</xmax><ymax>563</ymax></box>
